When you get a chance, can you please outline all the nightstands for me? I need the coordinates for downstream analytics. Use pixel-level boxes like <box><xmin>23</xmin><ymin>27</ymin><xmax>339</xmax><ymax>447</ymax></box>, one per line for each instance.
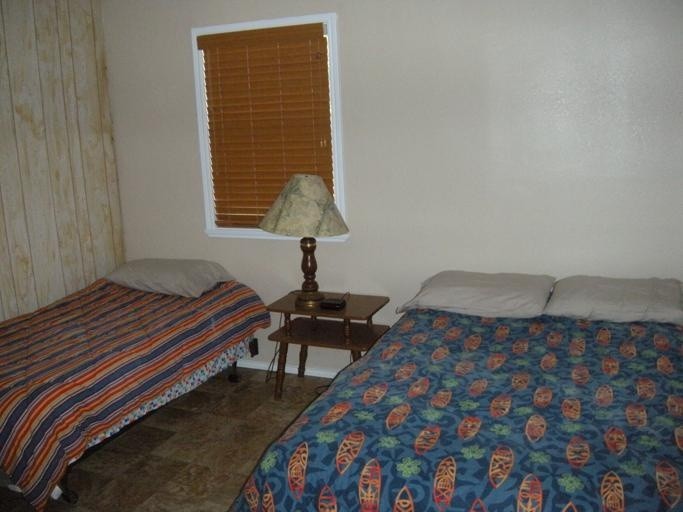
<box><xmin>262</xmin><ymin>289</ymin><xmax>392</xmax><ymax>400</ymax></box>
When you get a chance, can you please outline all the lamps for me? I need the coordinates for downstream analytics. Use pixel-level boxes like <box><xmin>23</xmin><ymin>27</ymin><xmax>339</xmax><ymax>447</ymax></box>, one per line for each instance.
<box><xmin>258</xmin><ymin>174</ymin><xmax>350</xmax><ymax>310</ymax></box>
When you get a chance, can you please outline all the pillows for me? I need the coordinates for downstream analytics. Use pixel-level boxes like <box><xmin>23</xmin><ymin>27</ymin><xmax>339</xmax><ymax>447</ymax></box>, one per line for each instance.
<box><xmin>396</xmin><ymin>271</ymin><xmax>682</xmax><ymax>327</ymax></box>
<box><xmin>104</xmin><ymin>257</ymin><xmax>235</xmax><ymax>298</ymax></box>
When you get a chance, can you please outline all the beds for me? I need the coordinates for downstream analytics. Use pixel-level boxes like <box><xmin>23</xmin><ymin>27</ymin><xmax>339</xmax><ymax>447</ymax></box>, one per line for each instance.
<box><xmin>0</xmin><ymin>273</ymin><xmax>269</xmax><ymax>504</ymax></box>
<box><xmin>256</xmin><ymin>290</ymin><xmax>682</xmax><ymax>512</ymax></box>
